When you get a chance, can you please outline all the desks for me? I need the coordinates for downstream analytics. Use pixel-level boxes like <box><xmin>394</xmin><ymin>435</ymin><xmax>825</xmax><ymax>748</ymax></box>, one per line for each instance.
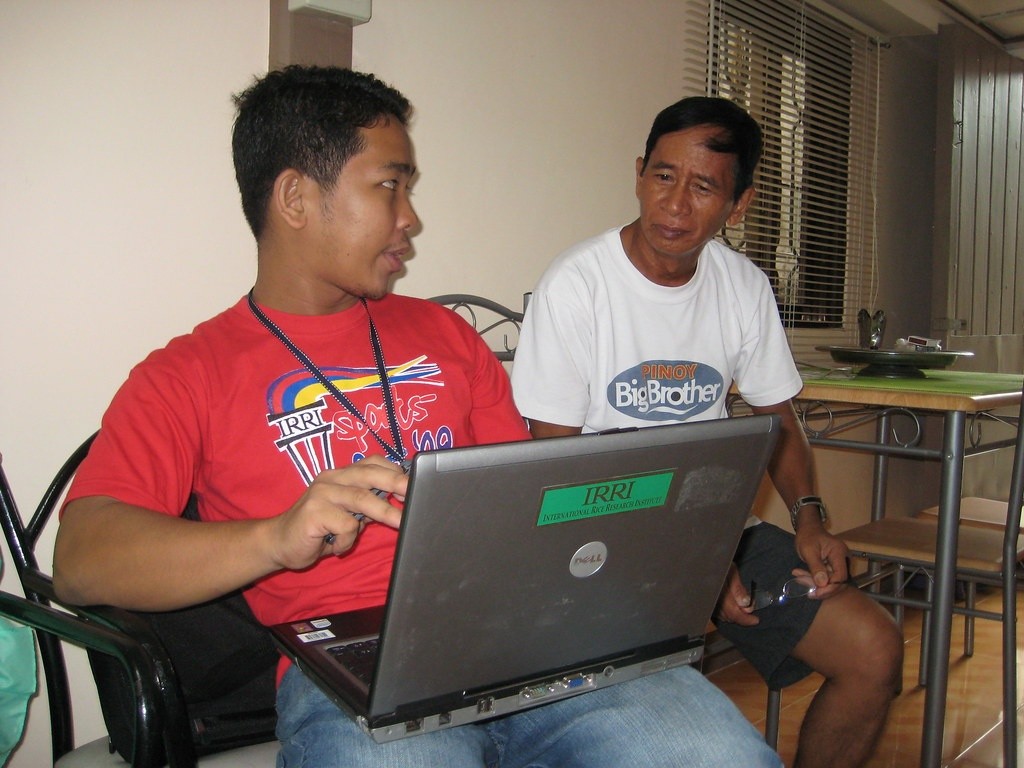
<box><xmin>724</xmin><ymin>370</ymin><xmax>1023</xmax><ymax>768</ymax></box>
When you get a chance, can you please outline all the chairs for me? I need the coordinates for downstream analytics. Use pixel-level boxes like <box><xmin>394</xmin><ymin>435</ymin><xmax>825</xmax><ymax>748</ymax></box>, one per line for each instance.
<box><xmin>0</xmin><ymin>428</ymin><xmax>281</xmax><ymax>768</ymax></box>
<box><xmin>828</xmin><ymin>383</ymin><xmax>1024</xmax><ymax>768</ymax></box>
<box><xmin>427</xmin><ymin>288</ymin><xmax>785</xmax><ymax>747</ymax></box>
<box><xmin>920</xmin><ymin>497</ymin><xmax>1024</xmax><ymax>689</ymax></box>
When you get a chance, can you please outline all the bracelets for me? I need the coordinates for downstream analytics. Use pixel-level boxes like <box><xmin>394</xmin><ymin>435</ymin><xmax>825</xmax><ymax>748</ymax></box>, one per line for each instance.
<box><xmin>791</xmin><ymin>495</ymin><xmax>827</xmax><ymax>530</ymax></box>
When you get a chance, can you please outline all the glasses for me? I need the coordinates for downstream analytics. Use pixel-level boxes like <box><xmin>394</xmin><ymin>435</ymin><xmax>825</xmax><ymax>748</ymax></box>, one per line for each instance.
<box><xmin>745</xmin><ymin>569</ymin><xmax>852</xmax><ymax>611</ymax></box>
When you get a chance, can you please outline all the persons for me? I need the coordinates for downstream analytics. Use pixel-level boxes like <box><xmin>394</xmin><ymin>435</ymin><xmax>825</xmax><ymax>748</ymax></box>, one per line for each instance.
<box><xmin>52</xmin><ymin>62</ymin><xmax>792</xmax><ymax>768</ymax></box>
<box><xmin>509</xmin><ymin>94</ymin><xmax>907</xmax><ymax>768</ymax></box>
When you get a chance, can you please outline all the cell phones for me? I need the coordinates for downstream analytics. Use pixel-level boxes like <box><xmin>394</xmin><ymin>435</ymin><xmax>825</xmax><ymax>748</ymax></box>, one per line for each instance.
<box><xmin>327</xmin><ymin>461</ymin><xmax>413</xmax><ymax>541</ymax></box>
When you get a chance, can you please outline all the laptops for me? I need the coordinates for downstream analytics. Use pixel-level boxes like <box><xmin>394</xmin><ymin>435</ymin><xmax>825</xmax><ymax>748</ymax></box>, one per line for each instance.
<box><xmin>269</xmin><ymin>414</ymin><xmax>784</xmax><ymax>745</ymax></box>
